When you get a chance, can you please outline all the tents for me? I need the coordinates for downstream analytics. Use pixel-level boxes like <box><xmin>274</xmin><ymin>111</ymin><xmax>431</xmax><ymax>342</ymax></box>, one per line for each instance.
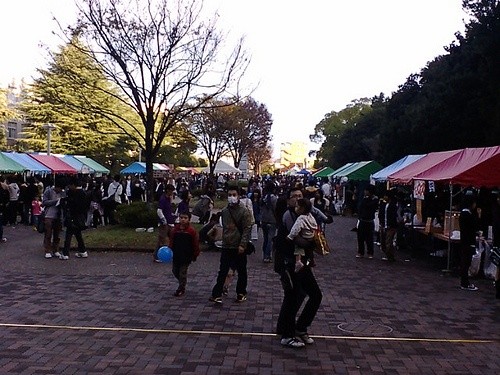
<box><xmin>203</xmin><ymin>160</ymin><xmax>243</xmax><ymax>174</ymax></box>
<box><xmin>119</xmin><ymin>162</ymin><xmax>210</xmax><ymax>175</ymax></box>
<box><xmin>326</xmin><ymin>145</ymin><xmax>500</xmax><ymax>274</ymax></box>
<box><xmin>0</xmin><ymin>151</ymin><xmax>110</xmax><ymax>186</ymax></box>
<box><xmin>311</xmin><ymin>166</ymin><xmax>336</xmax><ymax>177</ymax></box>
<box><xmin>296</xmin><ymin>168</ymin><xmax>311</xmax><ymax>174</ymax></box>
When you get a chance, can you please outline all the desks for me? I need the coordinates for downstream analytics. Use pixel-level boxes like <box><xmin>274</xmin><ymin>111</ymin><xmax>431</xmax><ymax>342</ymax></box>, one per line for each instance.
<box><xmin>407</xmin><ymin>226</ymin><xmax>465</xmax><ymax>274</ymax></box>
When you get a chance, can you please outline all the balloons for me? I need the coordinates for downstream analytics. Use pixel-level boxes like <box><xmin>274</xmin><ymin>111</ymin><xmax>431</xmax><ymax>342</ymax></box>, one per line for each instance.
<box><xmin>156</xmin><ymin>246</ymin><xmax>173</xmax><ymax>263</ymax></box>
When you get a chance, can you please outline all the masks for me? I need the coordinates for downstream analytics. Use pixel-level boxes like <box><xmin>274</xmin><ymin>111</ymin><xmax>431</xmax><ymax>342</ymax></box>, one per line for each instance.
<box><xmin>227</xmin><ymin>196</ymin><xmax>238</xmax><ymax>203</ymax></box>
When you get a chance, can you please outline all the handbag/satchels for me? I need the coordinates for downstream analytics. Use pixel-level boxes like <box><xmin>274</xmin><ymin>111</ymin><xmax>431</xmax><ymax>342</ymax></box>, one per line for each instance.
<box><xmin>271</xmin><ymin>235</ymin><xmax>288</xmax><ymax>274</ymax></box>
<box><xmin>244</xmin><ymin>240</ymin><xmax>256</xmax><ymax>255</ymax></box>
<box><xmin>192</xmin><ymin>197</ymin><xmax>211</xmax><ymax>218</ymax></box>
<box><xmin>102</xmin><ymin>193</ymin><xmax>115</xmax><ymax>206</ymax></box>
<box><xmin>251</xmin><ymin>224</ymin><xmax>259</xmax><ymax>240</ymax></box>
<box><xmin>468</xmin><ymin>240</ymin><xmax>482</xmax><ymax>278</ymax></box>
<box><xmin>37</xmin><ymin>211</ymin><xmax>45</xmax><ymax>233</ymax></box>
<box><xmin>207</xmin><ymin>224</ymin><xmax>223</xmax><ymax>241</ymax></box>
<box><xmin>314</xmin><ymin>230</ymin><xmax>330</xmax><ymax>255</ymax></box>
<box><xmin>484</xmin><ymin>247</ymin><xmax>500</xmax><ymax>288</ymax></box>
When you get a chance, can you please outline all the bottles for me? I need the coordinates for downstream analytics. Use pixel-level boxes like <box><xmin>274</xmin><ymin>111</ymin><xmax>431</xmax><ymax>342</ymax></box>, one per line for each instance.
<box><xmin>433</xmin><ymin>217</ymin><xmax>437</xmax><ymax>228</ymax></box>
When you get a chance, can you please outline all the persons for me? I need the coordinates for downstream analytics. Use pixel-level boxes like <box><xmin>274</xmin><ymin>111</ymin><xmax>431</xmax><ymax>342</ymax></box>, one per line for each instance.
<box><xmin>355</xmin><ymin>184</ymin><xmax>379</xmax><ymax>260</ymax></box>
<box><xmin>286</xmin><ymin>198</ymin><xmax>317</xmax><ymax>273</ymax></box>
<box><xmin>378</xmin><ymin>190</ymin><xmax>398</xmax><ymax>262</ymax></box>
<box><xmin>168</xmin><ymin>210</ymin><xmax>201</xmax><ymax>296</ymax></box>
<box><xmin>458</xmin><ymin>195</ymin><xmax>479</xmax><ymax>291</ymax></box>
<box><xmin>208</xmin><ymin>185</ymin><xmax>255</xmax><ymax>302</ymax></box>
<box><xmin>274</xmin><ymin>188</ymin><xmax>323</xmax><ymax>349</ymax></box>
<box><xmin>0</xmin><ymin>170</ymin><xmax>500</xmax><ymax>271</ymax></box>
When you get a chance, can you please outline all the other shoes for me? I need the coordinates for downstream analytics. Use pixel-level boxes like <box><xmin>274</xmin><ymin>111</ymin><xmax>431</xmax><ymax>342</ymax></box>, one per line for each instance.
<box><xmin>154</xmin><ymin>260</ymin><xmax>160</xmax><ymax>262</ymax></box>
<box><xmin>237</xmin><ymin>293</ymin><xmax>247</xmax><ymax>302</ymax></box>
<box><xmin>55</xmin><ymin>252</ymin><xmax>62</xmax><ymax>256</ymax></box>
<box><xmin>75</xmin><ymin>251</ymin><xmax>88</xmax><ymax>258</ymax></box>
<box><xmin>281</xmin><ymin>333</ymin><xmax>314</xmax><ymax>348</ymax></box>
<box><xmin>381</xmin><ymin>256</ymin><xmax>391</xmax><ymax>261</ymax></box>
<box><xmin>355</xmin><ymin>253</ymin><xmax>374</xmax><ymax>259</ymax></box>
<box><xmin>263</xmin><ymin>257</ymin><xmax>272</xmax><ymax>262</ymax></box>
<box><xmin>44</xmin><ymin>252</ymin><xmax>52</xmax><ymax>258</ymax></box>
<box><xmin>374</xmin><ymin>240</ymin><xmax>381</xmax><ymax>245</ymax></box>
<box><xmin>462</xmin><ymin>282</ymin><xmax>478</xmax><ymax>291</ymax></box>
<box><xmin>208</xmin><ymin>297</ymin><xmax>223</xmax><ymax>303</ymax></box>
<box><xmin>223</xmin><ymin>288</ymin><xmax>230</xmax><ymax>295</ymax></box>
<box><xmin>177</xmin><ymin>278</ymin><xmax>187</xmax><ymax>296</ymax></box>
<box><xmin>59</xmin><ymin>255</ymin><xmax>69</xmax><ymax>260</ymax></box>
<box><xmin>294</xmin><ymin>261</ymin><xmax>303</xmax><ymax>272</ymax></box>
